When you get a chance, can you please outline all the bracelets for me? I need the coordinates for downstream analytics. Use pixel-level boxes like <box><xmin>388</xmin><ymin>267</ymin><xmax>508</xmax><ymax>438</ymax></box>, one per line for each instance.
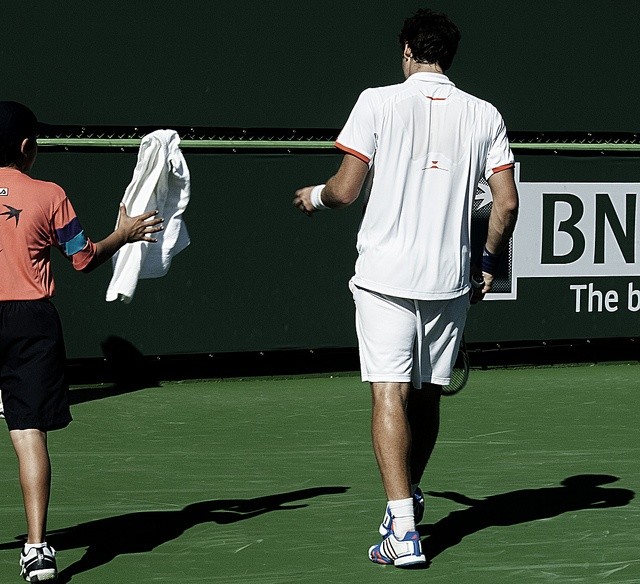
<box><xmin>311</xmin><ymin>184</ymin><xmax>332</xmax><ymax>211</ymax></box>
<box><xmin>481</xmin><ymin>245</ymin><xmax>496</xmax><ymax>278</ymax></box>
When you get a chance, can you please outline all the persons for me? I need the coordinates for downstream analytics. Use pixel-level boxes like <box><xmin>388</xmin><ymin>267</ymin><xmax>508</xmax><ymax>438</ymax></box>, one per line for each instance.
<box><xmin>1</xmin><ymin>100</ymin><xmax>165</xmax><ymax>584</ymax></box>
<box><xmin>294</xmin><ymin>17</ymin><xmax>520</xmax><ymax>569</ymax></box>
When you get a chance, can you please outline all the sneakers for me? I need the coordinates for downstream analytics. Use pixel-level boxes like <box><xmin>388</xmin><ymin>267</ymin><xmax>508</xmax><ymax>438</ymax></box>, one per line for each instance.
<box><xmin>18</xmin><ymin>541</ymin><xmax>58</xmax><ymax>584</ymax></box>
<box><xmin>367</xmin><ymin>529</ymin><xmax>427</xmax><ymax>567</ymax></box>
<box><xmin>378</xmin><ymin>487</ymin><xmax>425</xmax><ymax>536</ymax></box>
